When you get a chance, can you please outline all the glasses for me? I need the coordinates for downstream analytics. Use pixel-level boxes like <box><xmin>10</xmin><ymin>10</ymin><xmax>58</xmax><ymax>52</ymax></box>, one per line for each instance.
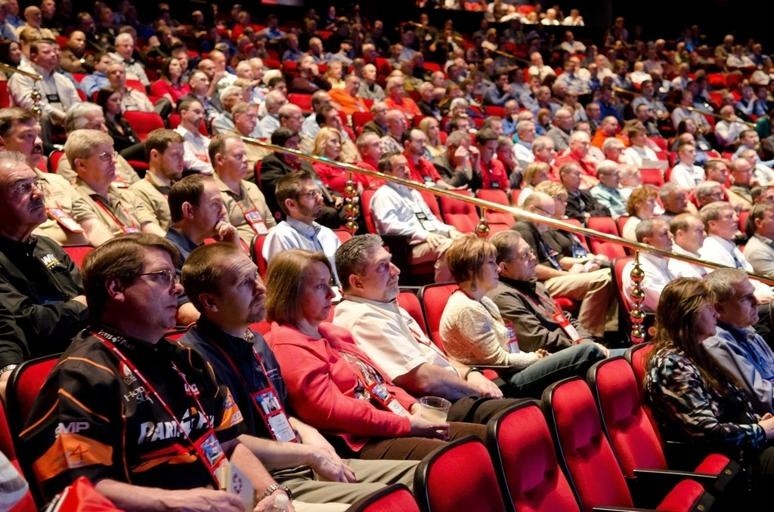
<box><xmin>298</xmin><ymin>189</ymin><xmax>324</xmax><ymax>198</ymax></box>
<box><xmin>137</xmin><ymin>267</ymin><xmax>183</xmax><ymax>285</ymax></box>
<box><xmin>96</xmin><ymin>151</ymin><xmax>118</xmax><ymax>161</ymax></box>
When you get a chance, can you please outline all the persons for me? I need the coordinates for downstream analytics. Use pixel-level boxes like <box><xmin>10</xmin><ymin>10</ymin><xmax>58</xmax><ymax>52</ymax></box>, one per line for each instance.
<box><xmin>1</xmin><ymin>1</ymin><xmax>504</xmax><ymax>511</ymax></box>
<box><xmin>442</xmin><ymin>4</ymin><xmax>774</xmax><ymax>512</ymax></box>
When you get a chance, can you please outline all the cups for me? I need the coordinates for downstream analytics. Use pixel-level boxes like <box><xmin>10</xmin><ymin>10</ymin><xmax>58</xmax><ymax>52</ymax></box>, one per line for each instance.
<box><xmin>419</xmin><ymin>394</ymin><xmax>452</xmax><ymax>420</ymax></box>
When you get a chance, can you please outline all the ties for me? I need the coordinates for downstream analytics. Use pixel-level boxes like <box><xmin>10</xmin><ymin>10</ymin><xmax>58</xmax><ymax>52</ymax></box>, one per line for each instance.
<box><xmin>537</xmin><ymin>240</ymin><xmax>564</xmax><ymax>273</ymax></box>
